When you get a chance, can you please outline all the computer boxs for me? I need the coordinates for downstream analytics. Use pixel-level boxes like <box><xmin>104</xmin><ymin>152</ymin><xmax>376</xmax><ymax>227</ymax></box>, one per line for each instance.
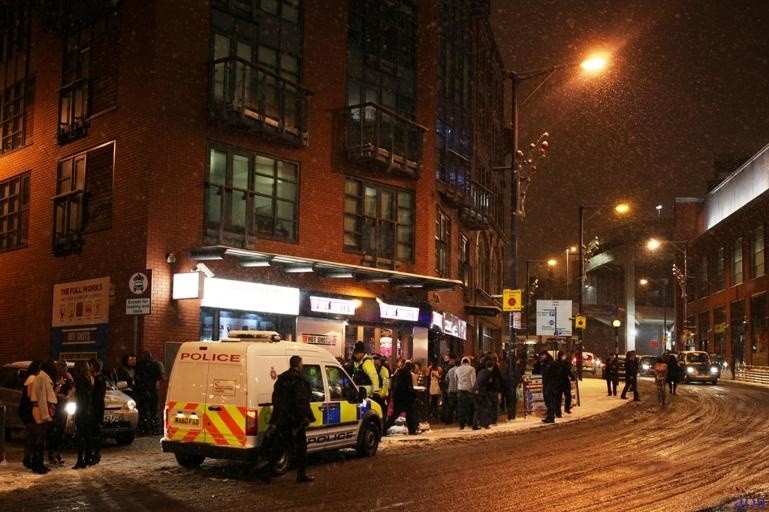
<box><xmin>435</xmin><ymin>294</ymin><xmax>440</xmax><ymax>304</ymax></box>
<box><xmin>197</xmin><ymin>263</ymin><xmax>215</xmax><ymax>278</ymax></box>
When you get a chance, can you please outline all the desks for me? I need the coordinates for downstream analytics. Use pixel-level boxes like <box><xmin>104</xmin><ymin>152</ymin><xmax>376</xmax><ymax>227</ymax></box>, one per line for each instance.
<box><xmin>566</xmin><ymin>245</ymin><xmax>578</xmax><ymax>301</ymax></box>
<box><xmin>612</xmin><ymin>318</ymin><xmax>620</xmax><ymax>386</ymax></box>
<box><xmin>525</xmin><ymin>257</ymin><xmax>558</xmax><ymax>340</ymax></box>
<box><xmin>646</xmin><ymin>237</ymin><xmax>688</xmax><ymax>353</ymax></box>
<box><xmin>640</xmin><ymin>277</ymin><xmax>668</xmax><ymax>351</ymax></box>
<box><xmin>509</xmin><ymin>48</ymin><xmax>613</xmax><ymax>372</ymax></box>
<box><xmin>577</xmin><ymin>199</ymin><xmax>631</xmax><ymax>361</ymax></box>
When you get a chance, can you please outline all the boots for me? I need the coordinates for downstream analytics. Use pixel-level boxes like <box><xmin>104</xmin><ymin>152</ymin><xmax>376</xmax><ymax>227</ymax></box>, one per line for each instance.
<box><xmin>1</xmin><ymin>359</ymin><xmax>139</xmax><ymax>452</ymax></box>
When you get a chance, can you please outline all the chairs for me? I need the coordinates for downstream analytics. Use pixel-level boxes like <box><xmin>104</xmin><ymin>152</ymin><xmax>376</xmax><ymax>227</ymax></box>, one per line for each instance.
<box><xmin>23</xmin><ymin>453</ymin><xmax>100</xmax><ymax>474</ymax></box>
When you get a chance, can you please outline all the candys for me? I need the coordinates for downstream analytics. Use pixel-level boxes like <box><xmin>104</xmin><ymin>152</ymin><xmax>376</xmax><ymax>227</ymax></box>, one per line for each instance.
<box><xmin>159</xmin><ymin>331</ymin><xmax>384</xmax><ymax>474</ymax></box>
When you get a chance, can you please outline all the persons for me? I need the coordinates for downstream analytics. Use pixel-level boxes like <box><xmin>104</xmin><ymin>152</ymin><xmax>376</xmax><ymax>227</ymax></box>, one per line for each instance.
<box><xmin>110</xmin><ymin>350</ymin><xmax>166</xmax><ymax>435</ymax></box>
<box><xmin>24</xmin><ymin>358</ymin><xmax>107</xmax><ymax>474</ymax></box>
<box><xmin>267</xmin><ymin>355</ymin><xmax>316</xmax><ymax>479</ymax></box>
<box><xmin>621</xmin><ymin>351</ymin><xmax>642</xmax><ymax>401</ymax></box>
<box><xmin>657</xmin><ymin>354</ymin><xmax>680</xmax><ymax>394</ymax></box>
<box><xmin>500</xmin><ymin>356</ymin><xmax>521</xmax><ymax>421</ymax></box>
<box><xmin>309</xmin><ymin>342</ymin><xmax>392</xmax><ymax>423</ymax></box>
<box><xmin>383</xmin><ymin>355</ymin><xmax>461</xmax><ymax>434</ymax></box>
<box><xmin>605</xmin><ymin>353</ymin><xmax>619</xmax><ymax>396</ymax></box>
<box><xmin>454</xmin><ymin>352</ymin><xmax>504</xmax><ymax>430</ymax></box>
<box><xmin>532</xmin><ymin>350</ymin><xmax>576</xmax><ymax>423</ymax></box>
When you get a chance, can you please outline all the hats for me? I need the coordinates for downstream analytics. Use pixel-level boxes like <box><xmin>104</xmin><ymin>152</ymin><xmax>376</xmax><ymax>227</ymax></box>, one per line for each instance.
<box><xmin>571</xmin><ymin>351</ymin><xmax>722</xmax><ymax>387</ymax></box>
